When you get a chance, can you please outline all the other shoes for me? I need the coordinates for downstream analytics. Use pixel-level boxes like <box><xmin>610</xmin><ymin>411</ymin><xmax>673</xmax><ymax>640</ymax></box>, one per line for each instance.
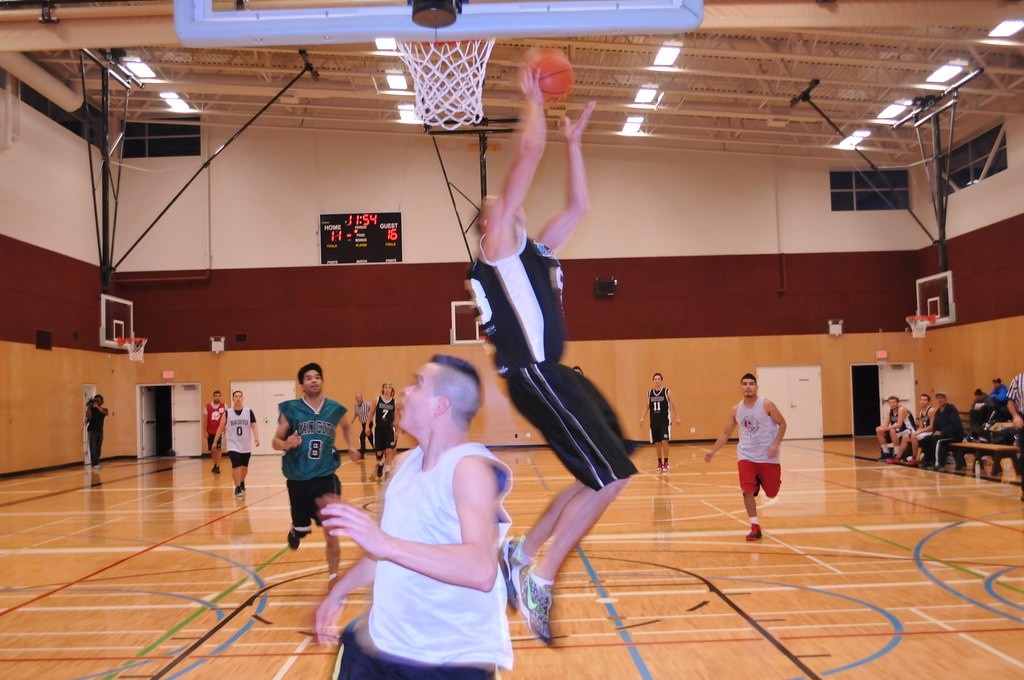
<box><xmin>288</xmin><ymin>527</ymin><xmax>300</xmax><ymax>549</ymax></box>
<box><xmin>377</xmin><ymin>463</ymin><xmax>384</xmax><ymax>477</ymax></box>
<box><xmin>211</xmin><ymin>463</ymin><xmax>221</xmax><ymax>473</ymax></box>
<box><xmin>92</xmin><ymin>464</ymin><xmax>101</xmax><ymax>468</ymax></box>
<box><xmin>656</xmin><ymin>462</ymin><xmax>663</xmax><ymax>471</ymax></box>
<box><xmin>991</xmin><ymin>463</ymin><xmax>1002</xmax><ymax>476</ymax></box>
<box><xmin>663</xmin><ymin>461</ymin><xmax>669</xmax><ymax>471</ymax></box>
<box><xmin>235</xmin><ymin>485</ymin><xmax>245</xmax><ymax>497</ymax></box>
<box><xmin>878</xmin><ymin>453</ymin><xmax>984</xmax><ymax>473</ymax></box>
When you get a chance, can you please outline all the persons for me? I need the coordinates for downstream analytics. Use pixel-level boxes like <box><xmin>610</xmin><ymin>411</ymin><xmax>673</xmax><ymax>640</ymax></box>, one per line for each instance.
<box><xmin>273</xmin><ymin>363</ymin><xmax>361</xmax><ymax>592</ymax></box>
<box><xmin>365</xmin><ymin>381</ymin><xmax>404</xmax><ymax>479</ymax></box>
<box><xmin>211</xmin><ymin>391</ymin><xmax>260</xmax><ymax>496</ymax></box>
<box><xmin>962</xmin><ymin>372</ymin><xmax>1024</xmax><ymax>500</ymax></box>
<box><xmin>467</xmin><ymin>62</ymin><xmax>635</xmax><ymax>652</ymax></box>
<box><xmin>875</xmin><ymin>396</ymin><xmax>917</xmax><ymax>462</ymax></box>
<box><xmin>351</xmin><ymin>394</ymin><xmax>377</xmax><ymax>459</ymax></box>
<box><xmin>917</xmin><ymin>392</ymin><xmax>963</xmax><ymax>471</ymax></box>
<box><xmin>203</xmin><ymin>391</ymin><xmax>228</xmax><ymax>473</ymax></box>
<box><xmin>317</xmin><ymin>355</ymin><xmax>514</xmax><ymax>680</ymax></box>
<box><xmin>86</xmin><ymin>395</ymin><xmax>108</xmax><ymax>468</ymax></box>
<box><xmin>639</xmin><ymin>373</ymin><xmax>682</xmax><ymax>470</ymax></box>
<box><xmin>885</xmin><ymin>395</ymin><xmax>935</xmax><ymax>466</ymax></box>
<box><xmin>705</xmin><ymin>373</ymin><xmax>787</xmax><ymax>541</ymax></box>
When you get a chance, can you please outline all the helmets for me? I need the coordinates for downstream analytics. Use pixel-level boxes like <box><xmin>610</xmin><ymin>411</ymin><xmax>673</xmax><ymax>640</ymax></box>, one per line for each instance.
<box><xmin>992</xmin><ymin>378</ymin><xmax>1001</xmax><ymax>384</ymax></box>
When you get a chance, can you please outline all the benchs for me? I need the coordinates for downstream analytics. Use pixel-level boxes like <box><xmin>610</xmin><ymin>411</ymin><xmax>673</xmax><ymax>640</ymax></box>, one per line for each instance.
<box><xmin>872</xmin><ymin>408</ymin><xmax>1024</xmax><ymax>477</ymax></box>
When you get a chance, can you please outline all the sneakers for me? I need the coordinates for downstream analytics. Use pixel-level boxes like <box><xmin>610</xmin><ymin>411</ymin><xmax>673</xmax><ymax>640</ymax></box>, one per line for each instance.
<box><xmin>498</xmin><ymin>535</ymin><xmax>535</xmax><ymax>613</ymax></box>
<box><xmin>746</xmin><ymin>524</ymin><xmax>761</xmax><ymax>540</ymax></box>
<box><xmin>753</xmin><ymin>475</ymin><xmax>761</xmax><ymax>496</ymax></box>
<box><xmin>512</xmin><ymin>561</ymin><xmax>553</xmax><ymax>645</ymax></box>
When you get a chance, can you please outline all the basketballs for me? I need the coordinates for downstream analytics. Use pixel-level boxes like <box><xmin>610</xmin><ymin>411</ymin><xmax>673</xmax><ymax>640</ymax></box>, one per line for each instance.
<box><xmin>530</xmin><ymin>49</ymin><xmax>576</xmax><ymax>102</ymax></box>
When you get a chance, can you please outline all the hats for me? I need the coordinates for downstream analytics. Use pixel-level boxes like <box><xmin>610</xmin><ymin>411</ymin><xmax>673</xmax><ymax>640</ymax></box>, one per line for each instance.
<box><xmin>935</xmin><ymin>392</ymin><xmax>947</xmax><ymax>398</ymax></box>
<box><xmin>975</xmin><ymin>389</ymin><xmax>984</xmax><ymax>394</ymax></box>
